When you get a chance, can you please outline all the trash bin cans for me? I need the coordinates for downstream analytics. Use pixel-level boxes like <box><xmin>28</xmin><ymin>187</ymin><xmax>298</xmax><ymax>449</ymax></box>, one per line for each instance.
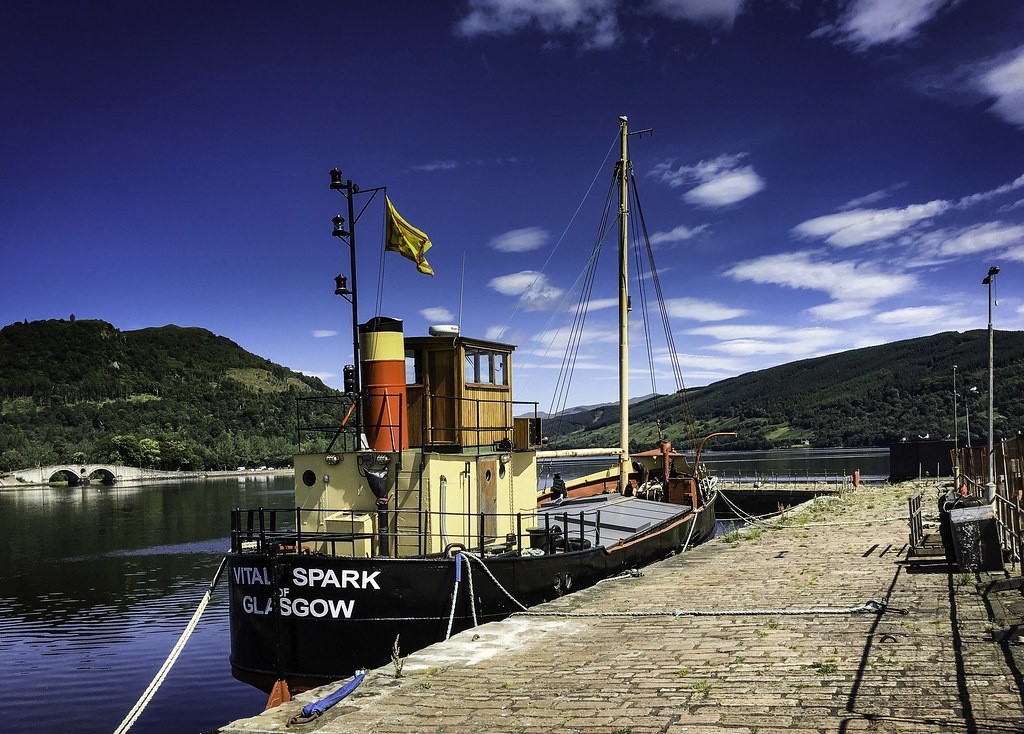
<box><xmin>939</xmin><ymin>495</ymin><xmax>988</xmax><ymax>565</ymax></box>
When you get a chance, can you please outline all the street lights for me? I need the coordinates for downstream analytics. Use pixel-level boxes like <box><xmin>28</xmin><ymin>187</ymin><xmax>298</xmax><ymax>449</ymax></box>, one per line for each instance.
<box><xmin>982</xmin><ymin>265</ymin><xmax>1001</xmax><ymax>484</ymax></box>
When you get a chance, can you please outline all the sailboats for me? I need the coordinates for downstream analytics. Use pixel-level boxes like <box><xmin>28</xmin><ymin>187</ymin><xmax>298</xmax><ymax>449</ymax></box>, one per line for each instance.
<box><xmin>221</xmin><ymin>113</ymin><xmax>726</xmax><ymax>698</ymax></box>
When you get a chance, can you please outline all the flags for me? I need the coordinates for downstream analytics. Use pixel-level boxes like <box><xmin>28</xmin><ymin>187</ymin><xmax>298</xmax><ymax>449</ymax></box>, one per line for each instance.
<box><xmin>383</xmin><ymin>194</ymin><xmax>435</xmax><ymax>277</ymax></box>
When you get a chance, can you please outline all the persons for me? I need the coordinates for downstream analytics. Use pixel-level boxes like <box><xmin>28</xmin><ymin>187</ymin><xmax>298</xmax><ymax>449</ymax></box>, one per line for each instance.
<box><xmin>551</xmin><ymin>473</ymin><xmax>567</xmax><ymax>498</ymax></box>
